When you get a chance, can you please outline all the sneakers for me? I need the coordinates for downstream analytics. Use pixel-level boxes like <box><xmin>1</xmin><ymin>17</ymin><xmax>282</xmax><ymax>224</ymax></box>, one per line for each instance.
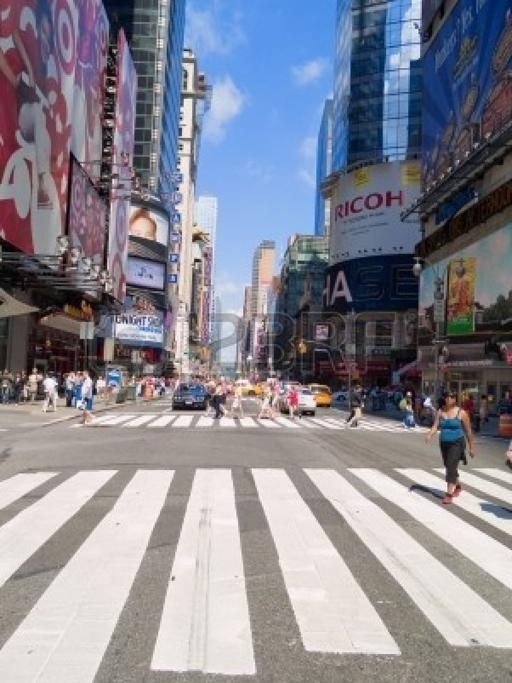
<box><xmin>442</xmin><ymin>485</ymin><xmax>462</xmax><ymax>504</ymax></box>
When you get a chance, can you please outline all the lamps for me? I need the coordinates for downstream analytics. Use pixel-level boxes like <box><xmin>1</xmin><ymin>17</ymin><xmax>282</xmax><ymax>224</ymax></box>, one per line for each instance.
<box><xmin>399</xmin><ymin>131</ymin><xmax>493</xmax><ymax>215</ymax></box>
<box><xmin>0</xmin><ymin>234</ymin><xmax>116</xmax><ymax>290</ymax></box>
<box><xmin>79</xmin><ymin>152</ymin><xmax>147</xmax><ymax>202</ymax></box>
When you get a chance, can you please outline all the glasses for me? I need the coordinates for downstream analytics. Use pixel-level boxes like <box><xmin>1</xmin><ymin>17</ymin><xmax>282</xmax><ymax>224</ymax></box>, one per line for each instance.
<box><xmin>443</xmin><ymin>394</ymin><xmax>456</xmax><ymax>398</ymax></box>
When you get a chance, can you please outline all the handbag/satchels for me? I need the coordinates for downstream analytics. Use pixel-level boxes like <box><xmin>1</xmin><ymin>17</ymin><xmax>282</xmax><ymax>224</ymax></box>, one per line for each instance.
<box><xmin>77</xmin><ymin>400</ymin><xmax>87</xmax><ymax>410</ymax></box>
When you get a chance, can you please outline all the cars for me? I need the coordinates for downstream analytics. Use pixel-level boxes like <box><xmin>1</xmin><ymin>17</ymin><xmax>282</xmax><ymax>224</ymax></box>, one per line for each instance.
<box><xmin>129</xmin><ymin>376</ymin><xmax>369</xmax><ymax>418</ymax></box>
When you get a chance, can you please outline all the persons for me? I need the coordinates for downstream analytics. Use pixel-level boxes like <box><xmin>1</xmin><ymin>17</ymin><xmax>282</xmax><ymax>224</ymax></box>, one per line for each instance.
<box><xmin>0</xmin><ymin>367</ymin><xmax>512</xmax><ymax>433</ymax></box>
<box><xmin>425</xmin><ymin>390</ymin><xmax>474</xmax><ymax>504</ymax></box>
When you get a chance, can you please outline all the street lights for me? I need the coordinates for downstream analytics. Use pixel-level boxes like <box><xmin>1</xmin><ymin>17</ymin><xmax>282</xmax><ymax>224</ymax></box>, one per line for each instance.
<box><xmin>406</xmin><ymin>252</ymin><xmax>467</xmax><ymax>427</ymax></box>
